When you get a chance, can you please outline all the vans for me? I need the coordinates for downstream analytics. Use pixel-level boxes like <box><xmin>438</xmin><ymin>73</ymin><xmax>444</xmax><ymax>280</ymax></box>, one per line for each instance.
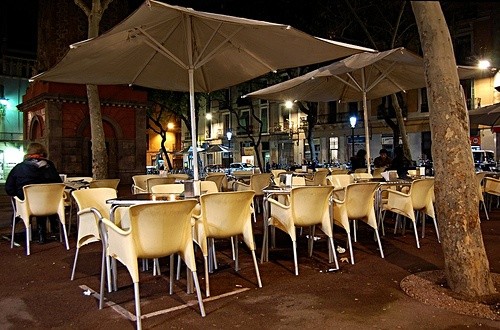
<box><xmin>471</xmin><ymin>145</ymin><xmax>497</xmax><ymax>171</ymax></box>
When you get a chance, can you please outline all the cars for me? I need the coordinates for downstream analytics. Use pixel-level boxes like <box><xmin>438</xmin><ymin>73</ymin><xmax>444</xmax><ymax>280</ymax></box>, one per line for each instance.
<box><xmin>204</xmin><ymin>162</ymin><xmax>257</xmax><ymax>174</ymax></box>
<box><xmin>146</xmin><ymin>165</ymin><xmax>156</xmax><ymax>173</ymax></box>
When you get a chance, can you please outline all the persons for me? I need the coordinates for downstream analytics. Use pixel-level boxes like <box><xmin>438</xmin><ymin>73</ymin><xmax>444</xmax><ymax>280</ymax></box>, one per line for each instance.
<box><xmin>348</xmin><ymin>149</ymin><xmax>367</xmax><ymax>175</ymax></box>
<box><xmin>266</xmin><ymin>162</ymin><xmax>270</xmax><ymax>173</ymax></box>
<box><xmin>5</xmin><ymin>143</ymin><xmax>64</xmax><ymax>244</ymax></box>
<box><xmin>379</xmin><ymin>146</ymin><xmax>412</xmax><ymax>179</ymax></box>
<box><xmin>373</xmin><ymin>149</ymin><xmax>392</xmax><ymax>168</ymax></box>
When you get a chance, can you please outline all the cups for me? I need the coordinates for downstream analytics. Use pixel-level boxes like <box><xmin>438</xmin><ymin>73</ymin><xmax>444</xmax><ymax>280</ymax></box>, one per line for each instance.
<box><xmin>389</xmin><ymin>171</ymin><xmax>397</xmax><ymax>182</ymax></box>
<box><xmin>343</xmin><ymin>164</ymin><xmax>346</xmax><ymax>169</ymax></box>
<box><xmin>415</xmin><ymin>167</ymin><xmax>425</xmax><ymax>180</ymax></box>
<box><xmin>308</xmin><ymin>169</ymin><xmax>313</xmax><ymax>174</ymax></box>
<box><xmin>340</xmin><ymin>165</ymin><xmax>344</xmax><ymax>170</ymax></box>
<box><xmin>162</xmin><ymin>171</ymin><xmax>167</xmax><ymax>177</ymax></box>
<box><xmin>59</xmin><ymin>174</ymin><xmax>67</xmax><ymax>183</ymax></box>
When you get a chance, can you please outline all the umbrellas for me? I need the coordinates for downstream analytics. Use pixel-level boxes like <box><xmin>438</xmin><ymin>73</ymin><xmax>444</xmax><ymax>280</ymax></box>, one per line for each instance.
<box><xmin>28</xmin><ymin>0</ymin><xmax>378</xmax><ymax>197</ymax></box>
<box><xmin>199</xmin><ymin>145</ymin><xmax>238</xmax><ymax>164</ymax></box>
<box><xmin>241</xmin><ymin>48</ymin><xmax>482</xmax><ymax>178</ymax></box>
<box><xmin>468</xmin><ymin>102</ymin><xmax>500</xmax><ymax>127</ymax></box>
<box><xmin>173</xmin><ymin>144</ymin><xmax>205</xmax><ymax>166</ymax></box>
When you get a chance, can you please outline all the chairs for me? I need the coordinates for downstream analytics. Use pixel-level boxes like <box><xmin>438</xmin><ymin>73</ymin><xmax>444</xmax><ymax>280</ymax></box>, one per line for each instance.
<box><xmin>475</xmin><ymin>169</ymin><xmax>500</xmax><ymax>219</ymax></box>
<box><xmin>10</xmin><ymin>167</ymin><xmax>440</xmax><ymax>330</ymax></box>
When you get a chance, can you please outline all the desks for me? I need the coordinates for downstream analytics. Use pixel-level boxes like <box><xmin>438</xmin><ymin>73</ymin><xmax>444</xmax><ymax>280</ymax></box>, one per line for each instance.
<box><xmin>261</xmin><ymin>185</ymin><xmax>325</xmax><ymax>248</ymax></box>
<box><xmin>106</xmin><ymin>192</ymin><xmax>199</xmax><ymax>294</ymax></box>
<box><xmin>355</xmin><ymin>178</ymin><xmax>414</xmax><ymax>184</ymax></box>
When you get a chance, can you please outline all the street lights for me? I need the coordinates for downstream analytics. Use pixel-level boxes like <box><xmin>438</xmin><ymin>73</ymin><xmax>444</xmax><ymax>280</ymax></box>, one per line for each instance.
<box><xmin>349</xmin><ymin>116</ymin><xmax>357</xmax><ymax>156</ymax></box>
<box><xmin>226</xmin><ymin>131</ymin><xmax>232</xmax><ymax>165</ymax></box>
